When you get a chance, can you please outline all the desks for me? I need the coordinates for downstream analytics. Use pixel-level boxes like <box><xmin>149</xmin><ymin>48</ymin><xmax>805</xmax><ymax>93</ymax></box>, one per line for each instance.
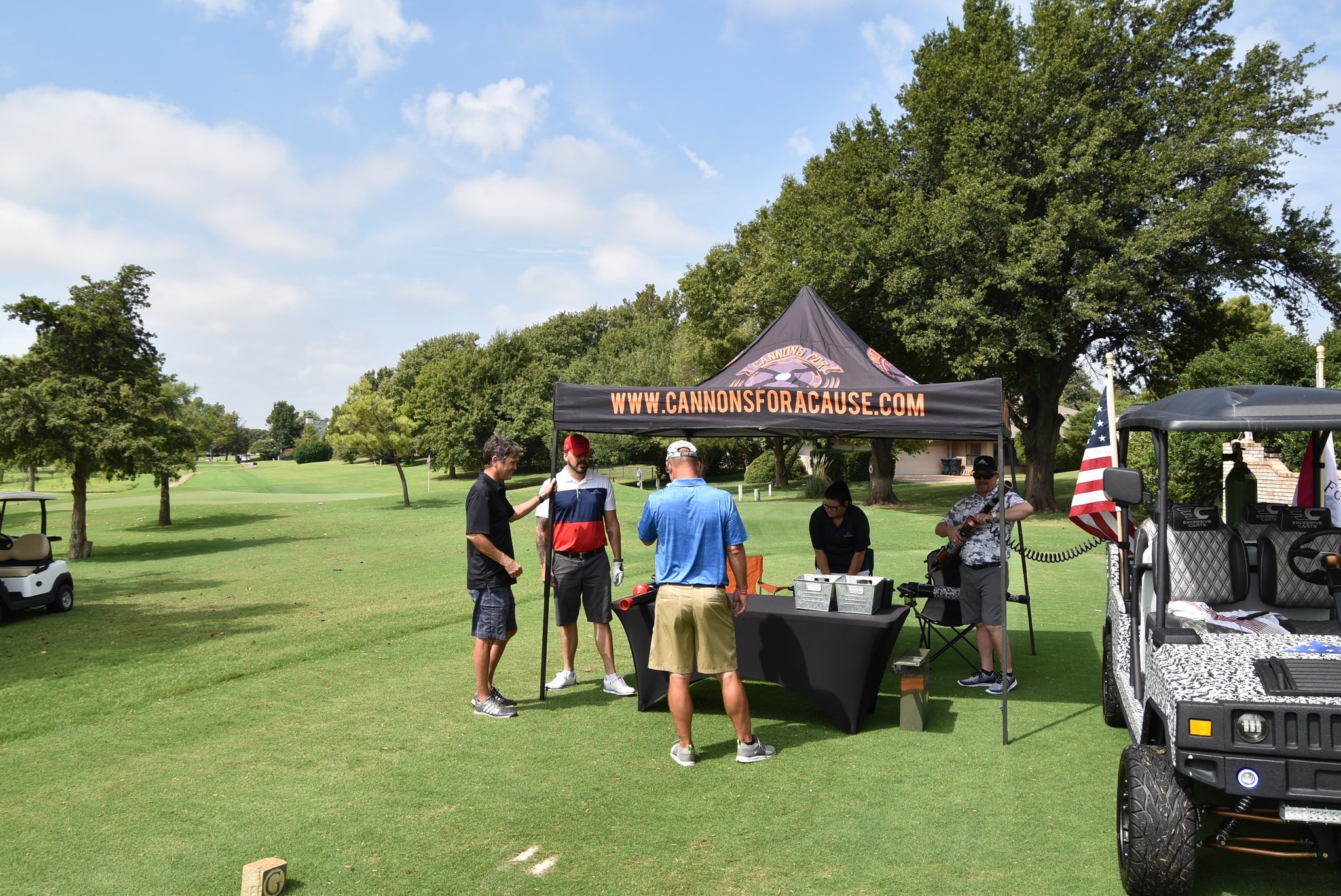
<box><xmin>611</xmin><ymin>593</ymin><xmax>910</xmax><ymax>735</ymax></box>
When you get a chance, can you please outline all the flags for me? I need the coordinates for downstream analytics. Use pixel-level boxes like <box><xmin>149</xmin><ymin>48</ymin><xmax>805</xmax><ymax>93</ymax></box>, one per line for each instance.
<box><xmin>1068</xmin><ymin>376</ymin><xmax>1136</xmax><ymax>558</ymax></box>
<box><xmin>1291</xmin><ymin>376</ymin><xmax>1341</xmax><ymax>529</ymax></box>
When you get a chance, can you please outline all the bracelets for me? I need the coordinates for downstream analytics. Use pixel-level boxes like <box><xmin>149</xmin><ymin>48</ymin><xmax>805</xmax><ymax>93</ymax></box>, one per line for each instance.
<box><xmin>537</xmin><ymin>494</ymin><xmax>544</xmax><ymax>504</ymax></box>
<box><xmin>613</xmin><ymin>558</ymin><xmax>624</xmax><ymax>563</ymax></box>
<box><xmin>735</xmin><ymin>587</ymin><xmax>747</xmax><ymax>595</ymax></box>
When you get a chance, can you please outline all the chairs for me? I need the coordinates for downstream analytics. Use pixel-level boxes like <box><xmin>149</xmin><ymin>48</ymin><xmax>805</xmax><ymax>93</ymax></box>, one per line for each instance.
<box><xmin>1132</xmin><ymin>500</ymin><xmax>1341</xmax><ymax>674</ymax></box>
<box><xmin>899</xmin><ymin>549</ymin><xmax>1010</xmax><ymax>674</ymax></box>
<box><xmin>725</xmin><ymin>555</ymin><xmax>789</xmax><ymax>595</ymax></box>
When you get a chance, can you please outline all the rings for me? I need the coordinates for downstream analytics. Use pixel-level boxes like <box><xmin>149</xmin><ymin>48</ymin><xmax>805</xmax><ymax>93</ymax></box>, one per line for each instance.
<box><xmin>953</xmin><ymin>539</ymin><xmax>956</xmax><ymax>542</ymax></box>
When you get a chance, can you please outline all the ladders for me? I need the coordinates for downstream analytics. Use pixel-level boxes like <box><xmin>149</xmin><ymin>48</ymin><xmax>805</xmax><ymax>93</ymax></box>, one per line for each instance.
<box><xmin>960</xmin><ymin>469</ymin><xmax>971</xmax><ymax>476</ymax></box>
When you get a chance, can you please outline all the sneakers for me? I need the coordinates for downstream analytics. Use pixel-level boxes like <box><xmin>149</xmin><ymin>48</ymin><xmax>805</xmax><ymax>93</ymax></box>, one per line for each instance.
<box><xmin>471</xmin><ymin>683</ymin><xmax>516</xmax><ymax>707</ymax></box>
<box><xmin>670</xmin><ymin>743</ymin><xmax>696</xmax><ymax>766</ymax></box>
<box><xmin>957</xmin><ymin>671</ymin><xmax>995</xmax><ymax>686</ymax></box>
<box><xmin>474</xmin><ymin>695</ymin><xmax>517</xmax><ymax>719</ymax></box>
<box><xmin>985</xmin><ymin>672</ymin><xmax>1017</xmax><ymax>694</ymax></box>
<box><xmin>736</xmin><ymin>736</ymin><xmax>776</xmax><ymax>763</ymax></box>
<box><xmin>603</xmin><ymin>675</ymin><xmax>636</xmax><ymax>696</ymax></box>
<box><xmin>545</xmin><ymin>672</ymin><xmax>577</xmax><ymax>690</ymax></box>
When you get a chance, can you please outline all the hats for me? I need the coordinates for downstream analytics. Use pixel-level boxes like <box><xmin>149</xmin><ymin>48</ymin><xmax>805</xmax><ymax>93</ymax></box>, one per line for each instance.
<box><xmin>564</xmin><ymin>433</ymin><xmax>591</xmax><ymax>456</ymax></box>
<box><xmin>971</xmin><ymin>455</ymin><xmax>997</xmax><ymax>472</ymax></box>
<box><xmin>665</xmin><ymin>440</ymin><xmax>697</xmax><ymax>473</ymax></box>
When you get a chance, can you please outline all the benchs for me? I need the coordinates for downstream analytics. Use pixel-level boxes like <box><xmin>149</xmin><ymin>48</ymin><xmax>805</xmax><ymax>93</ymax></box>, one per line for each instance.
<box><xmin>0</xmin><ymin>534</ymin><xmax>50</xmax><ymax>577</ymax></box>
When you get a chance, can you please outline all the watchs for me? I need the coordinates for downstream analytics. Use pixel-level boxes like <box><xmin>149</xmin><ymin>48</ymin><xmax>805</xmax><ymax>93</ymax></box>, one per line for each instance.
<box><xmin>990</xmin><ymin>511</ymin><xmax>997</xmax><ymax>522</ymax></box>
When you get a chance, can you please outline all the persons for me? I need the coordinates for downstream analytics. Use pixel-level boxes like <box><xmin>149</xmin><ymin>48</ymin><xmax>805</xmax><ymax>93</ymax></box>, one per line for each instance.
<box><xmin>810</xmin><ymin>479</ymin><xmax>871</xmax><ymax>584</ymax></box>
<box><xmin>466</xmin><ymin>435</ymin><xmax>557</xmax><ymax>718</ymax></box>
<box><xmin>934</xmin><ymin>455</ymin><xmax>1033</xmax><ymax>696</ymax></box>
<box><xmin>639</xmin><ymin>440</ymin><xmax>776</xmax><ymax>766</ymax></box>
<box><xmin>536</xmin><ymin>433</ymin><xmax>638</xmax><ymax>697</ymax></box>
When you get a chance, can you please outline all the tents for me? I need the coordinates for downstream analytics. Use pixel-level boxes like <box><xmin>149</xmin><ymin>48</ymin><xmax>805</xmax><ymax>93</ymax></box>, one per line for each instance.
<box><xmin>538</xmin><ymin>285</ymin><xmax>1021</xmax><ymax>745</ymax></box>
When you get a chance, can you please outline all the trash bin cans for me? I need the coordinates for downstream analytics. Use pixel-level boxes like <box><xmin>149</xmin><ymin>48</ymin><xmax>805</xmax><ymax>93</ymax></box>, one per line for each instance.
<box><xmin>940</xmin><ymin>458</ymin><xmax>962</xmax><ymax>475</ymax></box>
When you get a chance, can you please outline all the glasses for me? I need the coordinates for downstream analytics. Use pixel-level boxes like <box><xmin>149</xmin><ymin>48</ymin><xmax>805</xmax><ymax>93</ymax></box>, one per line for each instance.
<box><xmin>972</xmin><ymin>472</ymin><xmax>998</xmax><ymax>480</ymax></box>
<box><xmin>822</xmin><ymin>500</ymin><xmax>843</xmax><ymax>512</ymax></box>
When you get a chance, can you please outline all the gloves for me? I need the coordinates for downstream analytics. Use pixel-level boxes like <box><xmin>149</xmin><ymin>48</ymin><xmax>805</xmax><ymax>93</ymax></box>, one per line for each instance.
<box><xmin>612</xmin><ymin>559</ymin><xmax>624</xmax><ymax>587</ymax></box>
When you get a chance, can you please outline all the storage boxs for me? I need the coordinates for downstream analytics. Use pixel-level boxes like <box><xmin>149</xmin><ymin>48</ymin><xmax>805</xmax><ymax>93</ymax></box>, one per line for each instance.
<box><xmin>793</xmin><ymin>574</ymin><xmax>885</xmax><ymax>615</ymax></box>
